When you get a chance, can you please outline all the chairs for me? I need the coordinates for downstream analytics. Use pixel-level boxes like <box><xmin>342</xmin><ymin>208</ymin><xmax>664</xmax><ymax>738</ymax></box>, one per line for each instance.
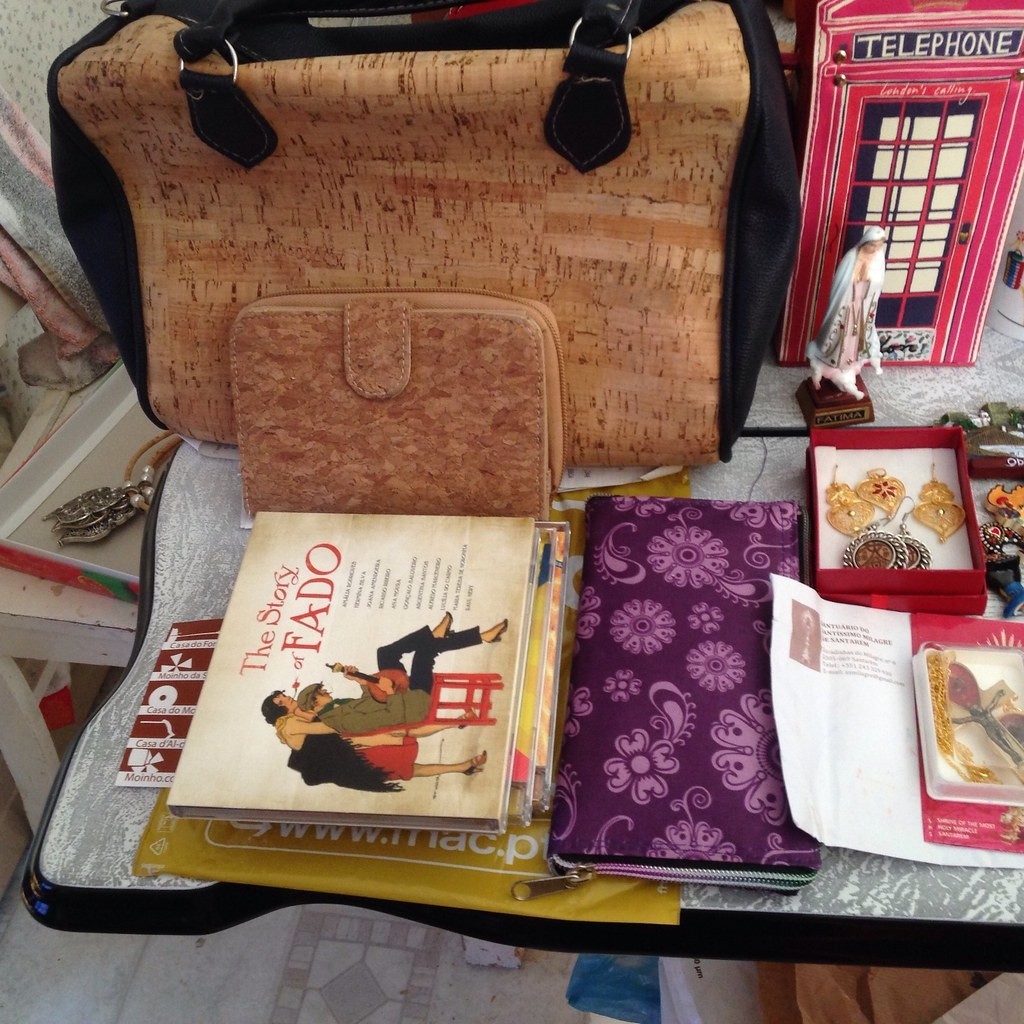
<box><xmin>0</xmin><ymin>380</ymin><xmax>534</xmax><ymax>968</ymax></box>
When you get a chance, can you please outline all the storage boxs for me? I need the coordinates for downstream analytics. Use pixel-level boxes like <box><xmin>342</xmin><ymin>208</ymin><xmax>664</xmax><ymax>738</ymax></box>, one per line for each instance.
<box><xmin>806</xmin><ymin>423</ymin><xmax>986</xmax><ymax>616</ymax></box>
<box><xmin>0</xmin><ymin>360</ymin><xmax>165</xmax><ymax>607</ymax></box>
<box><xmin>912</xmin><ymin>642</ymin><xmax>1024</xmax><ymax>807</ymax></box>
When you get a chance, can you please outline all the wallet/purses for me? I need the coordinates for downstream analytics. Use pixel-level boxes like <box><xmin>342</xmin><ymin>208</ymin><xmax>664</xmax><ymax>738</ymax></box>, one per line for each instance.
<box><xmin>229</xmin><ymin>288</ymin><xmax>571</xmax><ymax>522</ymax></box>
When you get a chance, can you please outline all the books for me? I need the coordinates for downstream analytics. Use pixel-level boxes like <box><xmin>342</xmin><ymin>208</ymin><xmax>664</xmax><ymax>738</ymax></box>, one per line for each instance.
<box><xmin>166</xmin><ymin>511</ymin><xmax>571</xmax><ymax>833</ymax></box>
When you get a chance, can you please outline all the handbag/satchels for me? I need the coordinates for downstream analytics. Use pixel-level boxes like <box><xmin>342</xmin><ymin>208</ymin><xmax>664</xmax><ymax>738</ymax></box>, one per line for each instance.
<box><xmin>46</xmin><ymin>0</ymin><xmax>800</xmax><ymax>468</ymax></box>
<box><xmin>511</xmin><ymin>496</ymin><xmax>822</xmax><ymax>901</ymax></box>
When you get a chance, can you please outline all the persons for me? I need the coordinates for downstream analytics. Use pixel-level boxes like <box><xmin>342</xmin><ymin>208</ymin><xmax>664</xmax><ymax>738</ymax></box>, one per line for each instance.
<box><xmin>816</xmin><ymin>227</ymin><xmax>885</xmax><ymax>372</ymax></box>
